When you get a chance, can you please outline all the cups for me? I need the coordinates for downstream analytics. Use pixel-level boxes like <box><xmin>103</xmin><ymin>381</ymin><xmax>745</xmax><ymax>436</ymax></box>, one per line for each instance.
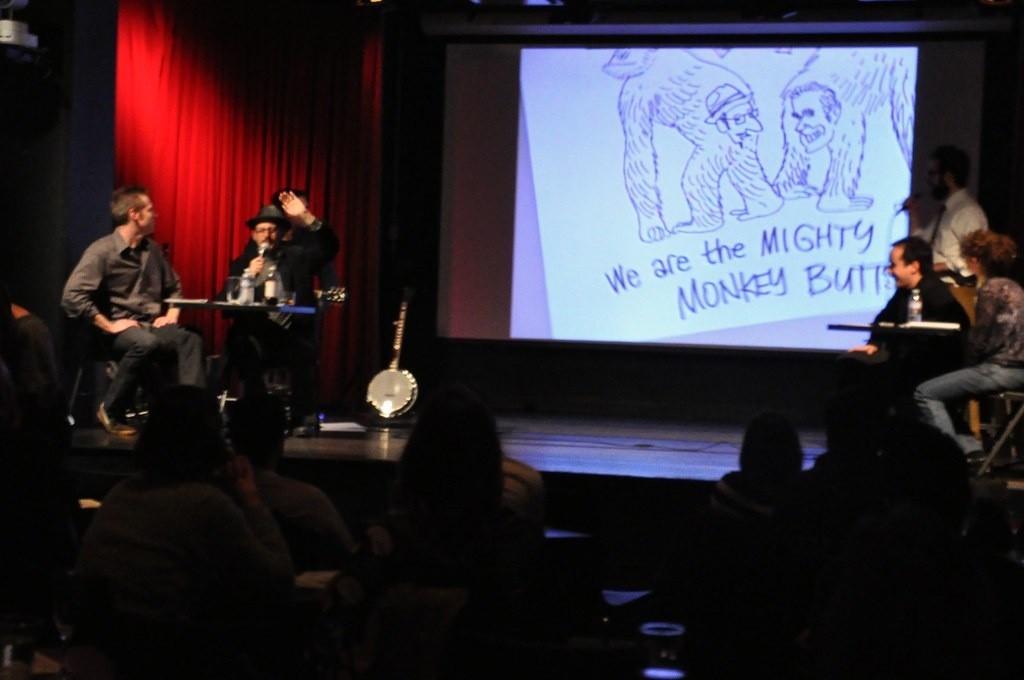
<box><xmin>279</xmin><ymin>291</ymin><xmax>295</xmax><ymax>305</ymax></box>
<box><xmin>641</xmin><ymin>622</ymin><xmax>684</xmax><ymax>678</ymax></box>
<box><xmin>0</xmin><ymin>616</ymin><xmax>42</xmax><ymax>680</ymax></box>
<box><xmin>225</xmin><ymin>277</ymin><xmax>239</xmax><ymax>303</ymax></box>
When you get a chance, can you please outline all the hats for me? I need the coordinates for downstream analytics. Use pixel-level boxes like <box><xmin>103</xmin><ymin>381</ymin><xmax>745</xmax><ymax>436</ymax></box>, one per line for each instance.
<box><xmin>245</xmin><ymin>205</ymin><xmax>292</xmax><ymax>231</ymax></box>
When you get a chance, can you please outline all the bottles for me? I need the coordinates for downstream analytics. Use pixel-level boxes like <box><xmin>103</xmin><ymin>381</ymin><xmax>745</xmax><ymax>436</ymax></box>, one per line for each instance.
<box><xmin>906</xmin><ymin>288</ymin><xmax>922</xmax><ymax>322</ymax></box>
<box><xmin>239</xmin><ymin>268</ymin><xmax>254</xmax><ymax>303</ymax></box>
<box><xmin>264</xmin><ymin>267</ymin><xmax>278</xmax><ymax>305</ymax></box>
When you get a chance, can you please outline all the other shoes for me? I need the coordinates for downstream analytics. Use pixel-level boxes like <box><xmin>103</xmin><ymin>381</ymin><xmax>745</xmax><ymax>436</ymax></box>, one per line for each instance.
<box><xmin>96</xmin><ymin>404</ymin><xmax>138</xmax><ymax>435</ymax></box>
<box><xmin>292</xmin><ymin>423</ymin><xmax>321</xmax><ymax>436</ymax></box>
<box><xmin>965</xmin><ymin>450</ymin><xmax>987</xmax><ymax>463</ymax></box>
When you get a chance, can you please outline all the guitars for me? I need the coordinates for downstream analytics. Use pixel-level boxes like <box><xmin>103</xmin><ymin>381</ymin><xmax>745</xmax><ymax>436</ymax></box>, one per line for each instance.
<box><xmin>364</xmin><ymin>299</ymin><xmax>420</xmax><ymax>421</ymax></box>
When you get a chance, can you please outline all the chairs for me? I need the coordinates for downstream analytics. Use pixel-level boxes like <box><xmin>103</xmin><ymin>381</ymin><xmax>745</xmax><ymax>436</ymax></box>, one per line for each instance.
<box><xmin>967</xmin><ymin>390</ymin><xmax>1023</xmax><ymax>475</ymax></box>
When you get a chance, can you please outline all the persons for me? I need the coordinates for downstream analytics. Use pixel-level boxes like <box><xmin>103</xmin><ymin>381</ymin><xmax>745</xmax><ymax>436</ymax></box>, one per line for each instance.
<box><xmin>222</xmin><ymin>190</ymin><xmax>340</xmax><ymax>437</ymax></box>
<box><xmin>841</xmin><ymin>236</ymin><xmax>971</xmax><ymax>385</ymax></box>
<box><xmin>902</xmin><ymin>144</ymin><xmax>988</xmax><ymax>327</ymax></box>
<box><xmin>688</xmin><ymin>381</ymin><xmax>1024</xmax><ymax>680</ymax></box>
<box><xmin>0</xmin><ymin>301</ymin><xmax>546</xmax><ymax>680</ymax></box>
<box><xmin>61</xmin><ymin>185</ymin><xmax>206</xmax><ymax>436</ymax></box>
<box><xmin>914</xmin><ymin>230</ymin><xmax>1024</xmax><ymax>472</ymax></box>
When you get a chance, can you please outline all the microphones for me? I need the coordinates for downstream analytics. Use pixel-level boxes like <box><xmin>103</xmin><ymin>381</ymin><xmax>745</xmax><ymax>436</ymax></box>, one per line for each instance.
<box><xmin>897</xmin><ymin>192</ymin><xmax>923</xmax><ymax>215</ymax></box>
<box><xmin>252</xmin><ymin>242</ymin><xmax>270</xmax><ymax>281</ymax></box>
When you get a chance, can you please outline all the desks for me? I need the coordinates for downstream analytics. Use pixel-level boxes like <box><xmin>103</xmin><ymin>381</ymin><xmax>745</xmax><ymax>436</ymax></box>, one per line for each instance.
<box><xmin>830</xmin><ymin>315</ymin><xmax>974</xmax><ymax>415</ymax></box>
<box><xmin>164</xmin><ymin>296</ymin><xmax>317</xmax><ymax>435</ymax></box>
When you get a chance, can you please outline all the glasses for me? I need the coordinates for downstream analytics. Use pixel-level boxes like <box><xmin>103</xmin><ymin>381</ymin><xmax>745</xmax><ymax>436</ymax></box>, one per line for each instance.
<box><xmin>923</xmin><ymin>168</ymin><xmax>938</xmax><ymax>178</ymax></box>
<box><xmin>254</xmin><ymin>228</ymin><xmax>276</xmax><ymax>233</ymax></box>
